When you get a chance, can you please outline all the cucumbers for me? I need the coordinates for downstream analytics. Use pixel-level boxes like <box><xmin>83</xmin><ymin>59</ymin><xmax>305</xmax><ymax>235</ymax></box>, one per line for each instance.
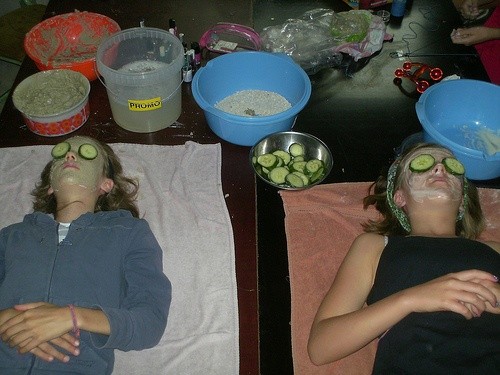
<box><xmin>79</xmin><ymin>143</ymin><xmax>98</xmax><ymax>160</ymax></box>
<box><xmin>442</xmin><ymin>157</ymin><xmax>465</xmax><ymax>175</ymax></box>
<box><xmin>409</xmin><ymin>154</ymin><xmax>435</xmax><ymax>173</ymax></box>
<box><xmin>257</xmin><ymin>143</ymin><xmax>326</xmax><ymax>188</ymax></box>
<box><xmin>51</xmin><ymin>141</ymin><xmax>71</xmax><ymax>158</ymax></box>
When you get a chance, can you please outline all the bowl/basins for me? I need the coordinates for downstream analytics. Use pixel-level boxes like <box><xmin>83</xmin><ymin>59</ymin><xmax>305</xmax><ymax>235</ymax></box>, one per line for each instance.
<box><xmin>249</xmin><ymin>131</ymin><xmax>333</xmax><ymax>191</ymax></box>
<box><xmin>191</xmin><ymin>51</ymin><xmax>311</xmax><ymax>147</ymax></box>
<box><xmin>415</xmin><ymin>79</ymin><xmax>500</xmax><ymax>180</ymax></box>
<box><xmin>24</xmin><ymin>12</ymin><xmax>121</xmax><ymax>81</ymax></box>
<box><xmin>13</xmin><ymin>69</ymin><xmax>90</xmax><ymax>137</ymax></box>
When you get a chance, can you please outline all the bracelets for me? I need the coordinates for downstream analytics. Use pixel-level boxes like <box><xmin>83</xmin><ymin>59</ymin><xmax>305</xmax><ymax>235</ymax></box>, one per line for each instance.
<box><xmin>66</xmin><ymin>304</ymin><xmax>79</xmax><ymax>338</ymax></box>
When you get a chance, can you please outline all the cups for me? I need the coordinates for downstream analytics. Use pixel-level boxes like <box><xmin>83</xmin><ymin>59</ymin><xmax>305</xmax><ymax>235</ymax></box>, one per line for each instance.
<box><xmin>377</xmin><ymin>10</ymin><xmax>390</xmax><ymax>23</ymax></box>
<box><xmin>391</xmin><ymin>0</ymin><xmax>406</xmax><ymax>16</ymax></box>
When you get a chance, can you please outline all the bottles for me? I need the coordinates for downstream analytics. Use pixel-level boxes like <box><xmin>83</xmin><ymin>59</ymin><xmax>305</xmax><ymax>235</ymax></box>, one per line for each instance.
<box><xmin>168</xmin><ymin>19</ymin><xmax>201</xmax><ymax>82</ymax></box>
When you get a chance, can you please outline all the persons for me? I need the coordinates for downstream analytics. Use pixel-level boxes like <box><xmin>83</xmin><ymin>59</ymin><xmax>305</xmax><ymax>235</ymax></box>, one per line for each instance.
<box><xmin>307</xmin><ymin>144</ymin><xmax>500</xmax><ymax>375</ymax></box>
<box><xmin>0</xmin><ymin>137</ymin><xmax>171</xmax><ymax>375</ymax></box>
<box><xmin>450</xmin><ymin>0</ymin><xmax>500</xmax><ymax>85</ymax></box>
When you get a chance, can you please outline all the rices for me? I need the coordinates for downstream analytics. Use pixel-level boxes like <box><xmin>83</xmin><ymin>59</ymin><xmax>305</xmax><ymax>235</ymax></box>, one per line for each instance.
<box><xmin>213</xmin><ymin>90</ymin><xmax>292</xmax><ymax>116</ymax></box>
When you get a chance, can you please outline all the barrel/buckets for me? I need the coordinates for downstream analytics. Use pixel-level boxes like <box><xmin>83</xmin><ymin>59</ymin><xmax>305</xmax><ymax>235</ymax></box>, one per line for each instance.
<box><xmin>94</xmin><ymin>28</ymin><xmax>188</xmax><ymax>133</ymax></box>
